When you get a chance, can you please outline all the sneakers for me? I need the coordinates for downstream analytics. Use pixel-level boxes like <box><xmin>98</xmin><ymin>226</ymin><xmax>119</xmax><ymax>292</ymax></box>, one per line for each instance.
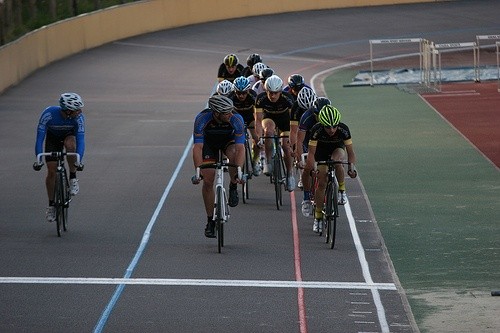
<box><xmin>313</xmin><ymin>218</ymin><xmax>323</xmax><ymax>232</ymax></box>
<box><xmin>262</xmin><ymin>159</ymin><xmax>273</xmax><ymax>174</ymax></box>
<box><xmin>298</xmin><ymin>179</ymin><xmax>303</xmax><ymax>187</ymax></box>
<box><xmin>287</xmin><ymin>176</ymin><xmax>295</xmax><ymax>192</ymax></box>
<box><xmin>302</xmin><ymin>199</ymin><xmax>311</xmax><ymax>217</ymax></box>
<box><xmin>204</xmin><ymin>216</ymin><xmax>215</xmax><ymax>239</ymax></box>
<box><xmin>338</xmin><ymin>190</ymin><xmax>347</xmax><ymax>204</ymax></box>
<box><xmin>69</xmin><ymin>177</ymin><xmax>80</xmax><ymax>196</ymax></box>
<box><xmin>46</xmin><ymin>207</ymin><xmax>56</xmax><ymax>221</ymax></box>
<box><xmin>229</xmin><ymin>183</ymin><xmax>239</xmax><ymax>208</ymax></box>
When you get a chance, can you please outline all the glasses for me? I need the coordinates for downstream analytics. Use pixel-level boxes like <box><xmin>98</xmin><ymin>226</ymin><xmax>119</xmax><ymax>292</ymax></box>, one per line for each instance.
<box><xmin>325</xmin><ymin>125</ymin><xmax>338</xmax><ymax>129</ymax></box>
<box><xmin>236</xmin><ymin>90</ymin><xmax>248</xmax><ymax>95</ymax></box>
<box><xmin>291</xmin><ymin>88</ymin><xmax>301</xmax><ymax>91</ymax></box>
<box><xmin>219</xmin><ymin>93</ymin><xmax>228</xmax><ymax>96</ymax></box>
<box><xmin>268</xmin><ymin>91</ymin><xmax>279</xmax><ymax>94</ymax></box>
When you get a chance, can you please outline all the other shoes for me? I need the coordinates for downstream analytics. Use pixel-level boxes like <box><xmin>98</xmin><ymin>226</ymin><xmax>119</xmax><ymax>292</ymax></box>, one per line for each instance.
<box><xmin>246</xmin><ymin>140</ymin><xmax>265</xmax><ymax>176</ymax></box>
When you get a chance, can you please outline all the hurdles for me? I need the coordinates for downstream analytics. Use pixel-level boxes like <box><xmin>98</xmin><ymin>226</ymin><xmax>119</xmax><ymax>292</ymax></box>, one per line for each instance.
<box><xmin>476</xmin><ymin>34</ymin><xmax>500</xmax><ymax>82</ymax></box>
<box><xmin>424</xmin><ymin>39</ymin><xmax>477</xmax><ymax>93</ymax></box>
<box><xmin>368</xmin><ymin>38</ymin><xmax>423</xmax><ymax>87</ymax></box>
<box><xmin>496</xmin><ymin>41</ymin><xmax>500</xmax><ymax>81</ymax></box>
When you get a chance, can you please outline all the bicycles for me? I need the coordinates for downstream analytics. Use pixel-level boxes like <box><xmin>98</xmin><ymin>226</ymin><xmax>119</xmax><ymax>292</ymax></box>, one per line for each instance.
<box><xmin>310</xmin><ymin>159</ymin><xmax>352</xmax><ymax>250</ymax></box>
<box><xmin>240</xmin><ymin>124</ymin><xmax>311</xmax><ymax>211</ymax></box>
<box><xmin>37</xmin><ymin>143</ymin><xmax>80</xmax><ymax>237</ymax></box>
<box><xmin>198</xmin><ymin>160</ymin><xmax>240</xmax><ymax>254</ymax></box>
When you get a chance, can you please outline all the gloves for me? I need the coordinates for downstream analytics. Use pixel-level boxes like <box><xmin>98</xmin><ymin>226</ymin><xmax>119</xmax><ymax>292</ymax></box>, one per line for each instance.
<box><xmin>32</xmin><ymin>162</ymin><xmax>45</xmax><ymax>172</ymax></box>
<box><xmin>75</xmin><ymin>163</ymin><xmax>85</xmax><ymax>171</ymax></box>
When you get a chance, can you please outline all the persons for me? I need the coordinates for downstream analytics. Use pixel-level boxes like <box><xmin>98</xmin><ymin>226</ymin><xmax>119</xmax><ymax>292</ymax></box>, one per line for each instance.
<box><xmin>33</xmin><ymin>92</ymin><xmax>85</xmax><ymax>222</ymax></box>
<box><xmin>254</xmin><ymin>74</ymin><xmax>296</xmax><ymax>192</ymax></box>
<box><xmin>218</xmin><ymin>53</ymin><xmax>245</xmax><ymax>84</ymax></box>
<box><xmin>242</xmin><ymin>53</ymin><xmax>262</xmax><ymax>78</ymax></box>
<box><xmin>227</xmin><ymin>75</ymin><xmax>262</xmax><ymax>177</ymax></box>
<box><xmin>191</xmin><ymin>94</ymin><xmax>246</xmax><ymax>238</ymax></box>
<box><xmin>305</xmin><ymin>104</ymin><xmax>358</xmax><ymax>233</ymax></box>
<box><xmin>288</xmin><ymin>86</ymin><xmax>318</xmax><ymax>189</ymax></box>
<box><xmin>281</xmin><ymin>73</ymin><xmax>317</xmax><ymax>168</ymax></box>
<box><xmin>251</xmin><ymin>67</ymin><xmax>275</xmax><ymax>167</ymax></box>
<box><xmin>246</xmin><ymin>62</ymin><xmax>268</xmax><ymax>90</ymax></box>
<box><xmin>296</xmin><ymin>96</ymin><xmax>333</xmax><ymax>217</ymax></box>
<box><xmin>211</xmin><ymin>79</ymin><xmax>237</xmax><ymax>102</ymax></box>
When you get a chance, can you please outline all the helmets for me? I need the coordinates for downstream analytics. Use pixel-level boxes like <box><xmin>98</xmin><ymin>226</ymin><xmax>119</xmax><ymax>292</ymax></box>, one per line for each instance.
<box><xmin>318</xmin><ymin>104</ymin><xmax>342</xmax><ymax>127</ymax></box>
<box><xmin>224</xmin><ymin>54</ymin><xmax>239</xmax><ymax>66</ymax></box>
<box><xmin>287</xmin><ymin>74</ymin><xmax>304</xmax><ymax>87</ymax></box>
<box><xmin>264</xmin><ymin>75</ymin><xmax>283</xmax><ymax>92</ymax></box>
<box><xmin>259</xmin><ymin>69</ymin><xmax>273</xmax><ymax>79</ymax></box>
<box><xmin>217</xmin><ymin>79</ymin><xmax>234</xmax><ymax>95</ymax></box>
<box><xmin>253</xmin><ymin>62</ymin><xmax>267</xmax><ymax>77</ymax></box>
<box><xmin>209</xmin><ymin>96</ymin><xmax>237</xmax><ymax>115</ymax></box>
<box><xmin>311</xmin><ymin>97</ymin><xmax>331</xmax><ymax>114</ymax></box>
<box><xmin>59</xmin><ymin>92</ymin><xmax>84</xmax><ymax>110</ymax></box>
<box><xmin>234</xmin><ymin>76</ymin><xmax>250</xmax><ymax>92</ymax></box>
<box><xmin>247</xmin><ymin>54</ymin><xmax>262</xmax><ymax>66</ymax></box>
<box><xmin>296</xmin><ymin>85</ymin><xmax>317</xmax><ymax>110</ymax></box>
